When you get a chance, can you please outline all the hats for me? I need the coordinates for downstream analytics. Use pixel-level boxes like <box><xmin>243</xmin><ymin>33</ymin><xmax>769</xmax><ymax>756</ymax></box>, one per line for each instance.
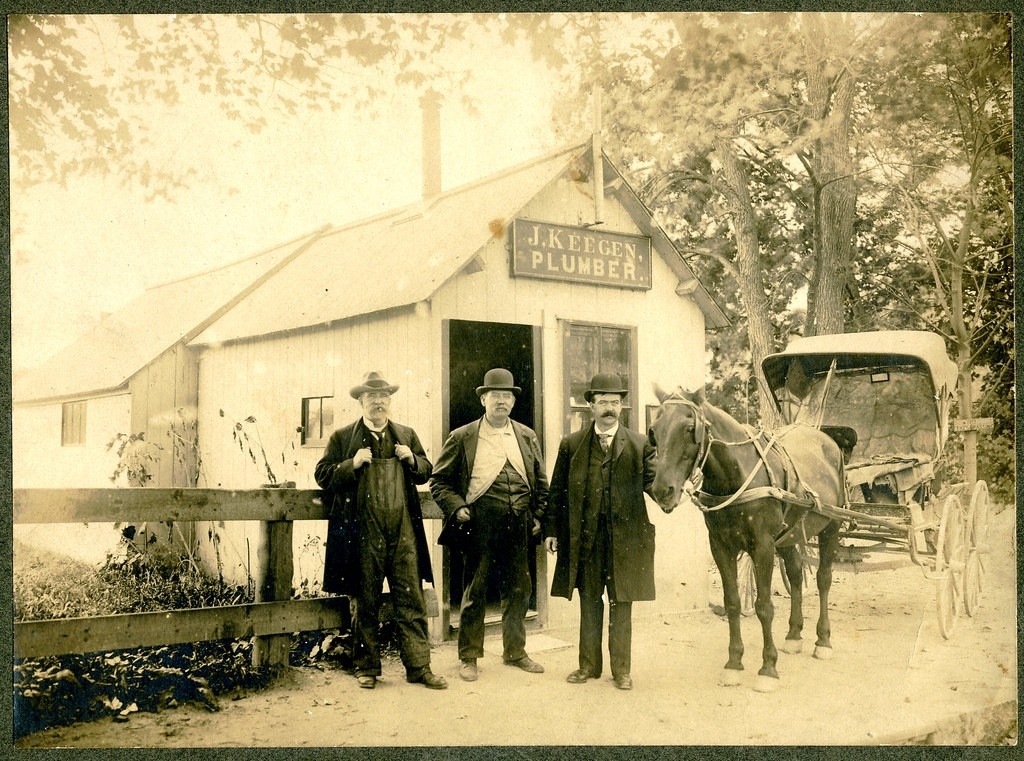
<box><xmin>348</xmin><ymin>370</ymin><xmax>399</xmax><ymax>399</ymax></box>
<box><xmin>475</xmin><ymin>368</ymin><xmax>522</xmax><ymax>397</ymax></box>
<box><xmin>583</xmin><ymin>372</ymin><xmax>629</xmax><ymax>403</ymax></box>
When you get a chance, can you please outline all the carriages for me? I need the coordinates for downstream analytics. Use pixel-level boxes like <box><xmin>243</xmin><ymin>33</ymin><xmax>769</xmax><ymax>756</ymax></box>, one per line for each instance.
<box><xmin>648</xmin><ymin>330</ymin><xmax>991</xmax><ymax>694</ymax></box>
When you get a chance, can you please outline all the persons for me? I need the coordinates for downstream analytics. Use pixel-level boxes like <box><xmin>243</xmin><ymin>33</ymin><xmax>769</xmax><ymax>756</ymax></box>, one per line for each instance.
<box><xmin>429</xmin><ymin>368</ymin><xmax>549</xmax><ymax>682</ymax></box>
<box><xmin>315</xmin><ymin>370</ymin><xmax>449</xmax><ymax>690</ymax></box>
<box><xmin>541</xmin><ymin>372</ymin><xmax>677</xmax><ymax>690</ymax></box>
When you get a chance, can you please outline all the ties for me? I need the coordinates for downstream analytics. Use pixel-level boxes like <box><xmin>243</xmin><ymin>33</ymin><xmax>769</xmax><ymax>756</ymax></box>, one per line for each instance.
<box><xmin>598</xmin><ymin>434</ymin><xmax>612</xmax><ymax>454</ymax></box>
<box><xmin>369</xmin><ymin>426</ymin><xmax>387</xmax><ymax>448</ymax></box>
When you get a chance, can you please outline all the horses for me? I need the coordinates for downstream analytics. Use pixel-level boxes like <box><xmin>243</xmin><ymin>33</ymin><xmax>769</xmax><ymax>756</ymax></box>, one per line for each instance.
<box><xmin>648</xmin><ymin>377</ymin><xmax>848</xmax><ymax>696</ymax></box>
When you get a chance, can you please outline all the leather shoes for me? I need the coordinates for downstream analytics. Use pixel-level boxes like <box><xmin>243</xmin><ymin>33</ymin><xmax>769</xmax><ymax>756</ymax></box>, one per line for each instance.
<box><xmin>612</xmin><ymin>673</ymin><xmax>633</xmax><ymax>689</ymax></box>
<box><xmin>416</xmin><ymin>672</ymin><xmax>449</xmax><ymax>689</ymax></box>
<box><xmin>502</xmin><ymin>655</ymin><xmax>545</xmax><ymax>673</ymax></box>
<box><xmin>357</xmin><ymin>674</ymin><xmax>376</xmax><ymax>688</ymax></box>
<box><xmin>457</xmin><ymin>657</ymin><xmax>478</xmax><ymax>681</ymax></box>
<box><xmin>566</xmin><ymin>668</ymin><xmax>601</xmax><ymax>683</ymax></box>
